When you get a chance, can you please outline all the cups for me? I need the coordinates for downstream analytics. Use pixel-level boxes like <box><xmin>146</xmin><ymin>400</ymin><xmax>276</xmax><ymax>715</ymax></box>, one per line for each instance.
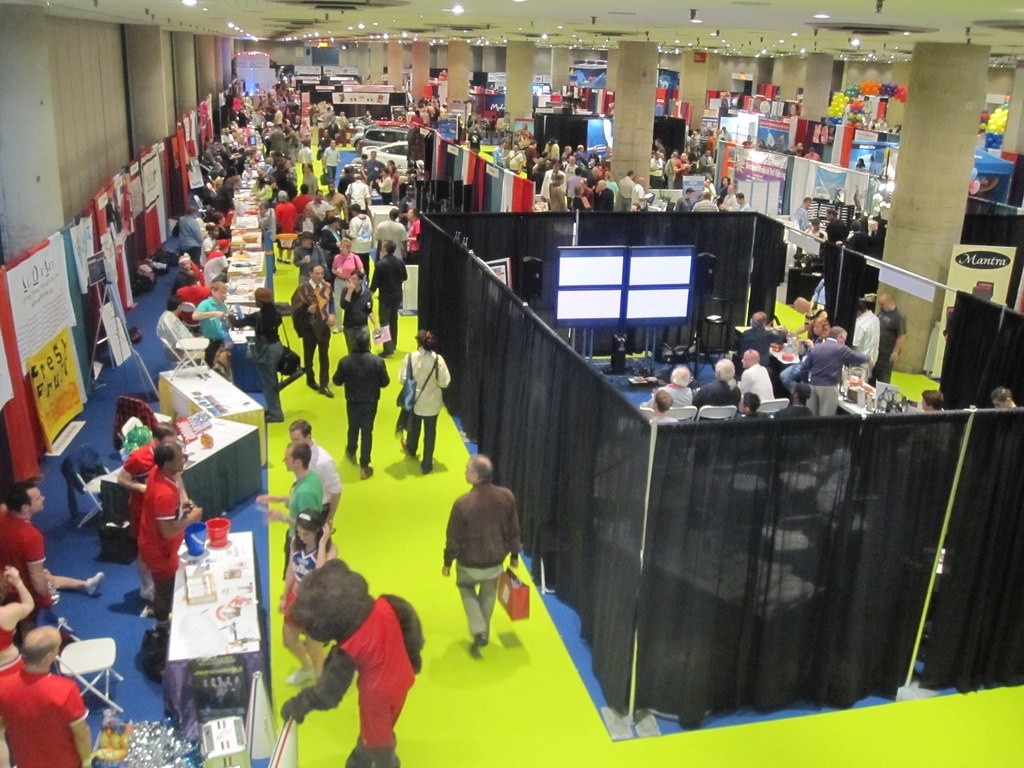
<box><xmin>305</xmin><ymin>255</ymin><xmax>311</xmax><ymax>263</ymax></box>
<box><xmin>337</xmin><ymin>268</ymin><xmax>342</xmax><ymax>276</ymax></box>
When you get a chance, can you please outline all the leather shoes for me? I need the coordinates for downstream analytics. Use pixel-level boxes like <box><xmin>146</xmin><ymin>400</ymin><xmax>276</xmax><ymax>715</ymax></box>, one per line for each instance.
<box><xmin>319</xmin><ymin>387</ymin><xmax>335</xmax><ymax>398</ymax></box>
<box><xmin>307</xmin><ymin>381</ymin><xmax>319</xmax><ymax>390</ymax></box>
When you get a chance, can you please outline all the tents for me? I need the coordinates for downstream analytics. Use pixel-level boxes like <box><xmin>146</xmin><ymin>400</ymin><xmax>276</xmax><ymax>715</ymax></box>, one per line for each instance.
<box><xmin>974</xmin><ymin>150</ymin><xmax>1015</xmax><ymax>203</ymax></box>
<box><xmin>849</xmin><ymin>140</ymin><xmax>898</xmax><ymax>180</ymax></box>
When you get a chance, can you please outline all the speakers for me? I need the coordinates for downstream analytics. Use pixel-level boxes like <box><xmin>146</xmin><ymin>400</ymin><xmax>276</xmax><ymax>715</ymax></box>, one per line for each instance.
<box><xmin>520</xmin><ymin>256</ymin><xmax>543</xmax><ymax>298</ymax></box>
<box><xmin>695</xmin><ymin>252</ymin><xmax>716</xmax><ymax>294</ymax></box>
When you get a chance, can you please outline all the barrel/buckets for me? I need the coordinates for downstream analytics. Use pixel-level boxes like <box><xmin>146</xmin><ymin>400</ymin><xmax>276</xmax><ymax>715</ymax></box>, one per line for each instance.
<box><xmin>184</xmin><ymin>522</ymin><xmax>206</xmax><ymax>556</ymax></box>
<box><xmin>205</xmin><ymin>517</ymin><xmax>231</xmax><ymax>547</ymax></box>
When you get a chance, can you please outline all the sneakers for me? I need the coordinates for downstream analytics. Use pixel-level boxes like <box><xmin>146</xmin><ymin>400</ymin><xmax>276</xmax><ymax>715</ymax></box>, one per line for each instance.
<box><xmin>85</xmin><ymin>572</ymin><xmax>104</xmax><ymax>595</ymax></box>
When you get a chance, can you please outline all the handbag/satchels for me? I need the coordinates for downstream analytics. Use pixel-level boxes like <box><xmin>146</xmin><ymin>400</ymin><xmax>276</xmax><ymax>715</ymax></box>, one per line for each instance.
<box><xmin>321</xmin><ymin>170</ymin><xmax>330</xmax><ymax>184</ymax></box>
<box><xmin>397</xmin><ymin>379</ymin><xmax>417</xmax><ymax>412</ymax></box>
<box><xmin>277</xmin><ymin>346</ymin><xmax>300</xmax><ymax>375</ymax></box>
<box><xmin>498</xmin><ymin>568</ymin><xmax>530</xmax><ymax>620</ymax></box>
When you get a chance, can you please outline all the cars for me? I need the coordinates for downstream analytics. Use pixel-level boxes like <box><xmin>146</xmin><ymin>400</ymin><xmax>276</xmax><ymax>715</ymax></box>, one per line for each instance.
<box><xmin>361</xmin><ymin>141</ymin><xmax>408</xmax><ymax>175</ymax></box>
<box><xmin>350</xmin><ymin>125</ymin><xmax>410</xmax><ymax>154</ymax></box>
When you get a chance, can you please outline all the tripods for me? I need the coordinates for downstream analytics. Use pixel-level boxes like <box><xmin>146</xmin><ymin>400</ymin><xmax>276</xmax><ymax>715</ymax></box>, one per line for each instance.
<box><xmin>666</xmin><ymin>294</ymin><xmax>715</xmax><ymax>381</ymax></box>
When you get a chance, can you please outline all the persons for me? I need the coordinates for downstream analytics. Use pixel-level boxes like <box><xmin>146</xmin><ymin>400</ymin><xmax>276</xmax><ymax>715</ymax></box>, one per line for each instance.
<box><xmin>158</xmin><ymin>72</ymin><xmax>452</xmax><ymax>479</ymax></box>
<box><xmin>992</xmin><ymin>387</ymin><xmax>1016</xmax><ymax>409</ymax></box>
<box><xmin>862</xmin><ymin>114</ymin><xmax>902</xmax><ymax>134</ymax></box>
<box><xmin>721</xmin><ymin>93</ymin><xmax>728</xmax><ymax>112</ymax></box>
<box><xmin>466</xmin><ymin>113</ymin><xmax>752</xmax><ymax>211</ymax></box>
<box><xmin>0</xmin><ymin>482</ymin><xmax>104</xmax><ymax>768</ymax></box>
<box><xmin>737</xmin><ymin>89</ymin><xmax>748</xmax><ymax>109</ymax></box>
<box><xmin>117</xmin><ymin>420</ymin><xmax>203</xmax><ymax>625</ymax></box>
<box><xmin>856</xmin><ymin>155</ymin><xmax>881</xmax><ymax>175</ymax></box>
<box><xmin>441</xmin><ymin>451</ymin><xmax>518</xmax><ymax>651</ymax></box>
<box><xmin>921</xmin><ymin>389</ymin><xmax>944</xmax><ymax>412</ymax></box>
<box><xmin>256</xmin><ymin>420</ymin><xmax>341</xmax><ymax>686</ymax></box>
<box><xmin>793</xmin><ymin>189</ymin><xmax>872</xmax><ymax>255</ymax></box>
<box><xmin>759</xmin><ymin>140</ymin><xmax>821</xmax><ymax>162</ymax></box>
<box><xmin>643</xmin><ymin>293</ymin><xmax>907</xmax><ymax>423</ymax></box>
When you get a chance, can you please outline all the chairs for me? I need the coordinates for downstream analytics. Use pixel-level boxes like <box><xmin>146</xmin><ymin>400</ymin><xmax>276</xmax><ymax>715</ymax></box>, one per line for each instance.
<box><xmin>164</xmin><ymin>311</ymin><xmax>209</xmax><ymax>369</ymax></box>
<box><xmin>194</xmin><ymin>195</ymin><xmax>215</xmax><ymax>219</ymax></box>
<box><xmin>63</xmin><ymin>442</ymin><xmax>112</xmax><ymax>529</ymax></box>
<box><xmin>55</xmin><ymin>618</ymin><xmax>124</xmax><ymax>713</ymax></box>
<box><xmin>117</xmin><ymin>395</ymin><xmax>171</xmax><ymax>446</ymax></box>
<box><xmin>641</xmin><ymin>399</ymin><xmax>789</xmax><ymax>423</ymax></box>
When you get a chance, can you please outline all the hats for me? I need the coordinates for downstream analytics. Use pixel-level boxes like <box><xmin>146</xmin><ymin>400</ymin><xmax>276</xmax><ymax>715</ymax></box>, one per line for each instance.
<box><xmin>298</xmin><ymin>232</ymin><xmax>314</xmax><ymax>241</ymax></box>
<box><xmin>324</xmin><ymin>205</ymin><xmax>336</xmax><ymax>213</ymax></box>
<box><xmin>255</xmin><ymin>288</ymin><xmax>273</xmax><ymax>303</ymax></box>
<box><xmin>278</xmin><ymin>190</ymin><xmax>288</xmax><ymax>201</ymax></box>
<box><xmin>327</xmin><ymin>216</ymin><xmax>339</xmax><ymax>223</ymax></box>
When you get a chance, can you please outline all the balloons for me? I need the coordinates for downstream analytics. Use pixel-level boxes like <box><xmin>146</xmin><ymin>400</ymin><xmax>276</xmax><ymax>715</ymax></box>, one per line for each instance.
<box><xmin>826</xmin><ymin>80</ymin><xmax>906</xmax><ymax>128</ymax></box>
<box><xmin>968</xmin><ymin>168</ymin><xmax>980</xmax><ymax>195</ymax></box>
<box><xmin>978</xmin><ymin>106</ymin><xmax>1008</xmax><ymax>149</ymax></box>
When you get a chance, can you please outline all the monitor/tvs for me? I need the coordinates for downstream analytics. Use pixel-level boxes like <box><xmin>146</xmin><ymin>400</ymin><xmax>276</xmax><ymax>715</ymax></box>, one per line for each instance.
<box><xmin>553</xmin><ymin>245</ymin><xmax>695</xmax><ymax>329</ymax></box>
<box><xmin>249</xmin><ymin>135</ymin><xmax>256</xmax><ymax>146</ymax></box>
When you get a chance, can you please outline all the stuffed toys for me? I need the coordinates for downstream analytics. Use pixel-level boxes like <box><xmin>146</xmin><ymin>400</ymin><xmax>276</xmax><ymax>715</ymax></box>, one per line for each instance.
<box><xmin>281</xmin><ymin>556</ymin><xmax>425</xmax><ymax>768</ymax></box>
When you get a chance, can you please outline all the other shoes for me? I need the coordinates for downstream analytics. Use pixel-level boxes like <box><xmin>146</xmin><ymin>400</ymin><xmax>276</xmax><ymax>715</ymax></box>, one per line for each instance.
<box><xmin>476</xmin><ymin>633</ymin><xmax>487</xmax><ymax>646</ymax></box>
<box><xmin>402</xmin><ymin>440</ymin><xmax>415</xmax><ymax>456</ymax></box>
<box><xmin>140</xmin><ymin>586</ymin><xmax>155</xmax><ymax>601</ymax></box>
<box><xmin>333</xmin><ymin>326</ymin><xmax>343</xmax><ymax>333</ymax></box>
<box><xmin>266</xmin><ymin>415</ymin><xmax>284</xmax><ymax>422</ymax></box>
<box><xmin>360</xmin><ymin>466</ymin><xmax>373</xmax><ymax>479</ymax></box>
<box><xmin>378</xmin><ymin>352</ymin><xmax>394</xmax><ymax>358</ymax></box>
<box><xmin>287</xmin><ymin>669</ymin><xmax>315</xmax><ymax>684</ymax></box>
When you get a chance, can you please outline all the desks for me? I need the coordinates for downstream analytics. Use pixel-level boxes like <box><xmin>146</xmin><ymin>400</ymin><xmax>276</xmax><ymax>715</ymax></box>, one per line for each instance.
<box><xmin>102</xmin><ymin>419</ymin><xmax>261</xmax><ymax>517</ymax></box>
<box><xmin>166</xmin><ymin>531</ymin><xmax>265</xmax><ymax>691</ymax></box>
<box><xmin>158</xmin><ymin>369</ymin><xmax>268</xmax><ymax>467</ymax></box>
<box><xmin>215</xmin><ymin>169</ymin><xmax>267</xmax><ymax>348</ymax></box>
